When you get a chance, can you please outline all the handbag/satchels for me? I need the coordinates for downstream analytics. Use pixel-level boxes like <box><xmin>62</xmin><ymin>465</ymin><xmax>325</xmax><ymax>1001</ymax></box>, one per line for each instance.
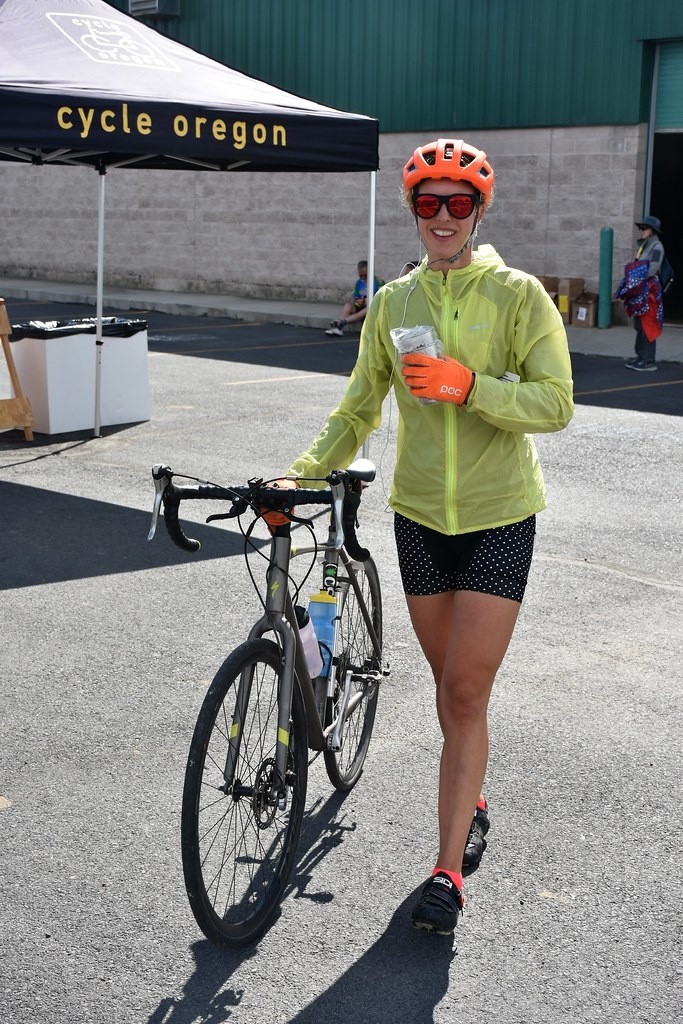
<box><xmin>660</xmin><ymin>258</ymin><xmax>674</xmax><ymax>292</ymax></box>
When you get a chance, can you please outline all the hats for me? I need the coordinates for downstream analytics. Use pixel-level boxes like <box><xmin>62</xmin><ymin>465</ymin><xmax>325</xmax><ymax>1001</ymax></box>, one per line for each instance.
<box><xmin>635</xmin><ymin>216</ymin><xmax>661</xmax><ymax>233</ymax></box>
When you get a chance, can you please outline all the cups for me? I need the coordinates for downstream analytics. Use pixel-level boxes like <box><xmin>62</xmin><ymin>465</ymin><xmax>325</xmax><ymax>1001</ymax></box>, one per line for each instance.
<box><xmin>393</xmin><ymin>326</ymin><xmax>442</xmax><ymax>406</ymax></box>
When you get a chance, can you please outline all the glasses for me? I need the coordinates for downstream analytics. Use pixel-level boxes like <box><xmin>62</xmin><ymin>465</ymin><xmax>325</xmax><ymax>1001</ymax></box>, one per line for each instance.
<box><xmin>639</xmin><ymin>225</ymin><xmax>648</xmax><ymax>231</ymax></box>
<box><xmin>411</xmin><ymin>188</ymin><xmax>478</xmax><ymax>220</ymax></box>
<box><xmin>360</xmin><ymin>274</ymin><xmax>367</xmax><ymax>278</ymax></box>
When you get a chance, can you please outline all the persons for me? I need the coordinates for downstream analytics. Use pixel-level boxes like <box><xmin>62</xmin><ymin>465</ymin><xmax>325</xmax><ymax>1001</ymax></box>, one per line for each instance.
<box><xmin>624</xmin><ymin>217</ymin><xmax>664</xmax><ymax>371</ymax></box>
<box><xmin>325</xmin><ymin>261</ymin><xmax>385</xmax><ymax>337</ymax></box>
<box><xmin>268</xmin><ymin>140</ymin><xmax>575</xmax><ymax>932</ymax></box>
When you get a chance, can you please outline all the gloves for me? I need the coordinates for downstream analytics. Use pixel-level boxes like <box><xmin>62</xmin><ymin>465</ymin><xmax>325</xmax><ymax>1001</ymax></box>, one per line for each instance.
<box><xmin>400</xmin><ymin>353</ymin><xmax>473</xmax><ymax>406</ymax></box>
<box><xmin>260</xmin><ymin>479</ymin><xmax>300</xmax><ymax>525</ymax></box>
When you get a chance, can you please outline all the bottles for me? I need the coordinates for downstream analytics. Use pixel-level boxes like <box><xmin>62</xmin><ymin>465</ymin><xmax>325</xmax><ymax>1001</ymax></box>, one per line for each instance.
<box><xmin>294</xmin><ymin>604</ymin><xmax>322</xmax><ymax>678</ymax></box>
<box><xmin>309</xmin><ymin>590</ymin><xmax>338</xmax><ymax>677</ymax></box>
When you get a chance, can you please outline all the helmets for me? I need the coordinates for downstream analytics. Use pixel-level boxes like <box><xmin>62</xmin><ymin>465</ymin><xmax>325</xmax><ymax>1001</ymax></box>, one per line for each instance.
<box><xmin>402</xmin><ymin>138</ymin><xmax>495</xmax><ymax>221</ymax></box>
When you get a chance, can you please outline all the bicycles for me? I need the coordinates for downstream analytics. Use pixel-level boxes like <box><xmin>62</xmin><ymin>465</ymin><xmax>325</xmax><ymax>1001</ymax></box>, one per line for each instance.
<box><xmin>146</xmin><ymin>458</ymin><xmax>393</xmax><ymax>954</ymax></box>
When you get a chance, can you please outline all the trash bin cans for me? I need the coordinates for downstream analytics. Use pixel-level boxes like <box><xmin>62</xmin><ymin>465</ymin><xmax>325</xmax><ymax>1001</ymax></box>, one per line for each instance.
<box><xmin>5</xmin><ymin>316</ymin><xmax>151</xmax><ymax>435</ymax></box>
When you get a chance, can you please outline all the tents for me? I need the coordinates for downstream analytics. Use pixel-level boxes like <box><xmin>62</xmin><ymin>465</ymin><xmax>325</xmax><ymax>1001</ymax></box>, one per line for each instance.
<box><xmin>1</xmin><ymin>0</ymin><xmax>380</xmax><ymax>487</ymax></box>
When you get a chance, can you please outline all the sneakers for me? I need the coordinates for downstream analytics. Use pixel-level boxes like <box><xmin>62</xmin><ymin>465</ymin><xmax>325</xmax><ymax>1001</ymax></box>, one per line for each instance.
<box><xmin>625</xmin><ymin>357</ymin><xmax>642</xmax><ymax>367</ymax></box>
<box><xmin>324</xmin><ymin>328</ymin><xmax>343</xmax><ymax>337</ymax></box>
<box><xmin>463</xmin><ymin>800</ymin><xmax>489</xmax><ymax>867</ymax></box>
<box><xmin>412</xmin><ymin>871</ymin><xmax>464</xmax><ymax>933</ymax></box>
<box><xmin>633</xmin><ymin>361</ymin><xmax>657</xmax><ymax>371</ymax></box>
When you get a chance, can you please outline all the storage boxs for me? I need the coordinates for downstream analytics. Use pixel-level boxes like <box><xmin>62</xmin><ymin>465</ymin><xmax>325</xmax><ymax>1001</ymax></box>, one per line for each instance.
<box><xmin>537</xmin><ymin>276</ymin><xmax>599</xmax><ymax>328</ymax></box>
